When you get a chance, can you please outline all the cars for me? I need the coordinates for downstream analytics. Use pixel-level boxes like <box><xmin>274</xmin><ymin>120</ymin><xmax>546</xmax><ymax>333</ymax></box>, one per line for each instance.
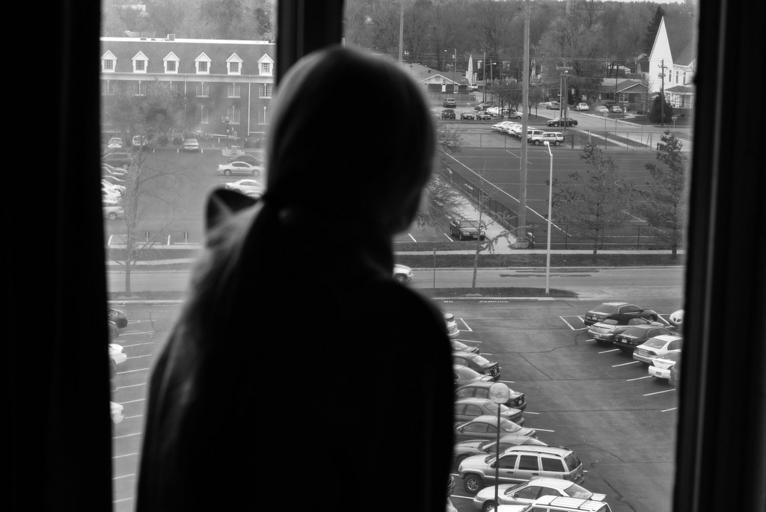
<box><xmin>107</xmin><ymin>320</ymin><xmax>119</xmax><ymax>341</ymax></box>
<box><xmin>183</xmin><ymin>138</ymin><xmax>199</xmax><ymax>151</ymax></box>
<box><xmin>217</xmin><ymin>148</ymin><xmax>264</xmax><ymax>204</ymax></box>
<box><xmin>584</xmin><ymin>302</ymin><xmax>658</xmax><ymax>326</ymax></box>
<box><xmin>108</xmin><ymin>358</ymin><xmax>118</xmax><ymax>377</ymax></box>
<box><xmin>545</xmin><ymin>101</ymin><xmax>560</xmax><ymax>110</ymax></box>
<box><xmin>460</xmin><ymin>112</ymin><xmax>476</xmax><ymax>121</ymax></box>
<box><xmin>450</xmin><ymin>217</ymin><xmax>485</xmax><ymax>242</ymax></box>
<box><xmin>109</xmin><ymin>401</ymin><xmax>124</xmax><ymax>425</ymax></box>
<box><xmin>612</xmin><ymin>324</ymin><xmax>682</xmax><ymax>350</ymax></box>
<box><xmin>547</xmin><ymin>118</ymin><xmax>578</xmax><ymax>128</ymax></box>
<box><xmin>393</xmin><ymin>261</ymin><xmax>414</xmax><ymax>285</ymax></box>
<box><xmin>99</xmin><ymin>137</ymin><xmax>128</xmax><ymax>220</ymax></box>
<box><xmin>595</xmin><ymin>105</ymin><xmax>610</xmax><ymax>114</ymax></box>
<box><xmin>452</xmin><ymin>435</ymin><xmax>548</xmax><ymax>466</ymax></box>
<box><xmin>632</xmin><ymin>334</ymin><xmax>683</xmax><ymax>364</ymax></box>
<box><xmin>106</xmin><ymin>306</ymin><xmax>128</xmax><ymax>327</ymax></box>
<box><xmin>442</xmin><ymin>313</ymin><xmax>538</xmax><ymax>442</ymax></box>
<box><xmin>472</xmin><ymin>477</ymin><xmax>607</xmax><ymax>511</ymax></box>
<box><xmin>108</xmin><ymin>343</ymin><xmax>127</xmax><ymax>365</ymax></box>
<box><xmin>648</xmin><ymin>348</ymin><xmax>682</xmax><ymax>379</ymax></box>
<box><xmin>612</xmin><ymin>105</ymin><xmax>622</xmax><ymax>114</ymax></box>
<box><xmin>587</xmin><ymin>314</ymin><xmax>664</xmax><ymax>345</ymax></box>
<box><xmin>670</xmin><ymin>308</ymin><xmax>685</xmax><ymax>326</ymax></box>
<box><xmin>474</xmin><ymin>102</ymin><xmax>523</xmax><ymax>121</ymax></box>
<box><xmin>576</xmin><ymin>103</ymin><xmax>590</xmax><ymax>112</ymax></box>
<box><xmin>491</xmin><ymin>121</ymin><xmax>564</xmax><ymax>147</ymax></box>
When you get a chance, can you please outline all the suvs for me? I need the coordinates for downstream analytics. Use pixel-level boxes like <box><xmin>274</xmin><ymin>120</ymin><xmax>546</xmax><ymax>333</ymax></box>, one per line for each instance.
<box><xmin>442</xmin><ymin>98</ymin><xmax>457</xmax><ymax>107</ymax></box>
<box><xmin>132</xmin><ymin>135</ymin><xmax>147</xmax><ymax>149</ymax></box>
<box><xmin>459</xmin><ymin>445</ymin><xmax>586</xmax><ymax>494</ymax></box>
<box><xmin>488</xmin><ymin>495</ymin><xmax>612</xmax><ymax>511</ymax></box>
<box><xmin>441</xmin><ymin>109</ymin><xmax>457</xmax><ymax>120</ymax></box>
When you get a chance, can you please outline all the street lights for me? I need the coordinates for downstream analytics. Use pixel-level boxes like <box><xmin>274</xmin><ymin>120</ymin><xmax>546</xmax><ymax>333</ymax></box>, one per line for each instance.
<box><xmin>564</xmin><ymin>70</ymin><xmax>569</xmax><ymax>128</ymax></box>
<box><xmin>540</xmin><ymin>140</ymin><xmax>554</xmax><ymax>295</ymax></box>
<box><xmin>671</xmin><ymin>116</ymin><xmax>678</xmax><ymax>135</ymax></box>
<box><xmin>483</xmin><ymin>62</ymin><xmax>496</xmax><ymax>86</ymax></box>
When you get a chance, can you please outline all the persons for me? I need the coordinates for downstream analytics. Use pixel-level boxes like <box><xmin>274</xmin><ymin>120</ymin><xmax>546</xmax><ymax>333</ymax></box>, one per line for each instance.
<box><xmin>133</xmin><ymin>41</ymin><xmax>459</xmax><ymax>509</ymax></box>
